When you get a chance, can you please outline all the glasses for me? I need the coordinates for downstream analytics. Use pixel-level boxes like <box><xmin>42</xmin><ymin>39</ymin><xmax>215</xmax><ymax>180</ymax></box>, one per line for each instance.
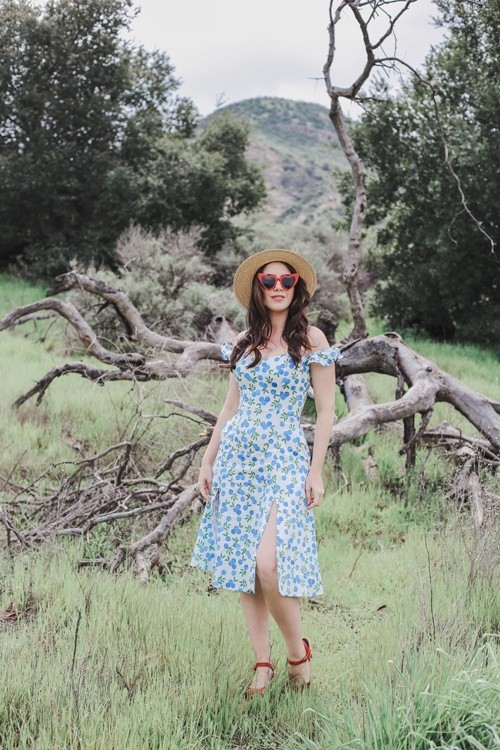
<box><xmin>258</xmin><ymin>273</ymin><xmax>299</xmax><ymax>290</ymax></box>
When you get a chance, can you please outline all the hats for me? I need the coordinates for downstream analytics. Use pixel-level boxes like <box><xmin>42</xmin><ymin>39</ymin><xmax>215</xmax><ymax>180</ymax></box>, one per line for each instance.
<box><xmin>233</xmin><ymin>249</ymin><xmax>317</xmax><ymax>310</ymax></box>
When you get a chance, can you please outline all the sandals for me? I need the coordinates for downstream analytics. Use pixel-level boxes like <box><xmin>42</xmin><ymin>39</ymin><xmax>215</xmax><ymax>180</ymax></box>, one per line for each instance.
<box><xmin>250</xmin><ymin>661</ymin><xmax>273</xmax><ymax>696</ymax></box>
<box><xmin>286</xmin><ymin>638</ymin><xmax>312</xmax><ymax>692</ymax></box>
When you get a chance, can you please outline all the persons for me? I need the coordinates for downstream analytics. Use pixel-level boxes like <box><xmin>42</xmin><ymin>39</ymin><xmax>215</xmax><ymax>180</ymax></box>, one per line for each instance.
<box><xmin>190</xmin><ymin>248</ymin><xmax>344</xmax><ymax>697</ymax></box>
